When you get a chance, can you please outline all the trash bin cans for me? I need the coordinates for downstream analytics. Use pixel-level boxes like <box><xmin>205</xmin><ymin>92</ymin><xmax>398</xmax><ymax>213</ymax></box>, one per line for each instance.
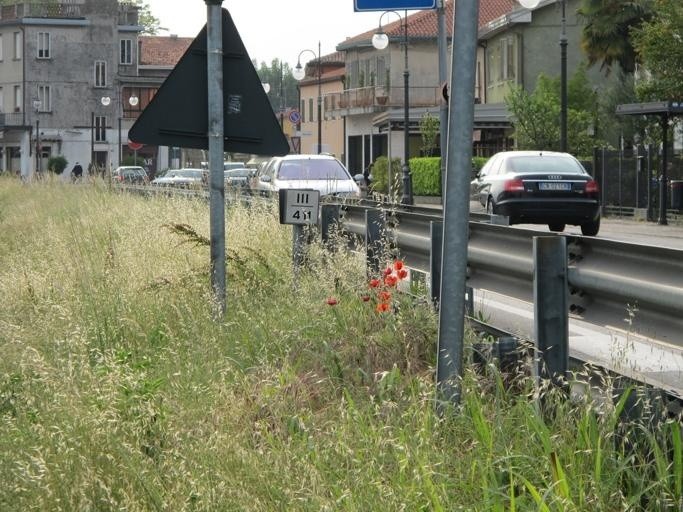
<box><xmin>671</xmin><ymin>180</ymin><xmax>683</xmax><ymax>214</ymax></box>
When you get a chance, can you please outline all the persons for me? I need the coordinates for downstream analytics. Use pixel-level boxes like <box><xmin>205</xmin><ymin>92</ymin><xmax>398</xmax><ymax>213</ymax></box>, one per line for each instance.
<box><xmin>363</xmin><ymin>162</ymin><xmax>375</xmax><ymax>201</ymax></box>
<box><xmin>71</xmin><ymin>161</ymin><xmax>82</xmax><ymax>184</ymax></box>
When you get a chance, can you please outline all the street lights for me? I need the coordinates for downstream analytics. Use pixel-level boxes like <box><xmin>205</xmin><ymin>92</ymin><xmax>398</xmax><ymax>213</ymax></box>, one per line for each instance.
<box><xmin>516</xmin><ymin>1</ymin><xmax>568</xmax><ymax>153</ymax></box>
<box><xmin>100</xmin><ymin>79</ymin><xmax>138</xmax><ymax>167</ymax></box>
<box><xmin>259</xmin><ymin>58</ymin><xmax>284</xmax><ymax>134</ymax></box>
<box><xmin>31</xmin><ymin>94</ymin><xmax>42</xmax><ymax>173</ymax></box>
<box><xmin>371</xmin><ymin>9</ymin><xmax>415</xmax><ymax>207</ymax></box>
<box><xmin>293</xmin><ymin>39</ymin><xmax>321</xmax><ymax>155</ymax></box>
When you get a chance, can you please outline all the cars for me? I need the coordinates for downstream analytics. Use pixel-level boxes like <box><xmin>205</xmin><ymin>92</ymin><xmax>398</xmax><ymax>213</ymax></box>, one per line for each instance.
<box><xmin>468</xmin><ymin>147</ymin><xmax>601</xmax><ymax>240</ymax></box>
<box><xmin>106</xmin><ymin>152</ymin><xmax>361</xmax><ymax>211</ymax></box>
